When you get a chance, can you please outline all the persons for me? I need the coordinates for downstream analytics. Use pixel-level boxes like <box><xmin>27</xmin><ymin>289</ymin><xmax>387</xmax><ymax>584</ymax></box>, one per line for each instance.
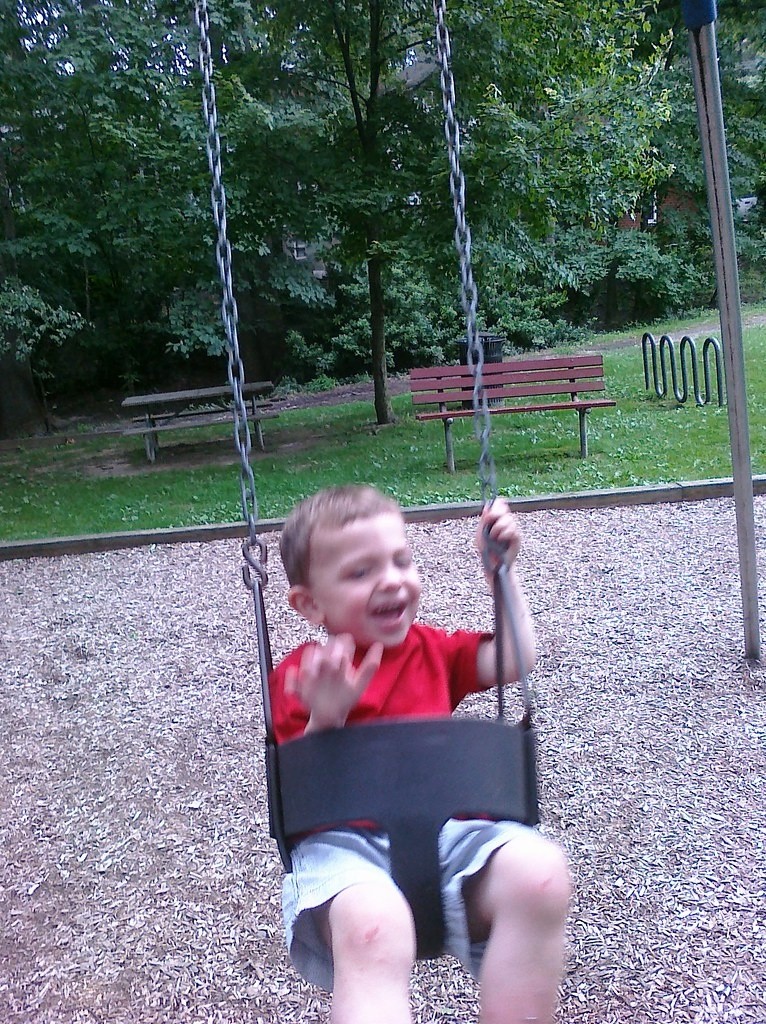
<box><xmin>263</xmin><ymin>484</ymin><xmax>573</xmax><ymax>1024</ymax></box>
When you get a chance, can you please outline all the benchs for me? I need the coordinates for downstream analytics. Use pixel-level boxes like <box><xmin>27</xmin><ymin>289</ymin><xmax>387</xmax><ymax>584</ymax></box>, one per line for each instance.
<box><xmin>410</xmin><ymin>354</ymin><xmax>617</xmax><ymax>472</ymax></box>
<box><xmin>123</xmin><ymin>401</ymin><xmax>279</xmax><ymax>463</ymax></box>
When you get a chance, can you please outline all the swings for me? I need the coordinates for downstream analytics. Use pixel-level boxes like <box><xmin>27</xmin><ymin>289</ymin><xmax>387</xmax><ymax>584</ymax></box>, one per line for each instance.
<box><xmin>194</xmin><ymin>0</ymin><xmax>542</xmax><ymax>967</ymax></box>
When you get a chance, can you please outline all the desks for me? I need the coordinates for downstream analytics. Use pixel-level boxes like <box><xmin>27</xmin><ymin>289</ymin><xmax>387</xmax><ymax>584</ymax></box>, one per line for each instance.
<box><xmin>121</xmin><ymin>381</ymin><xmax>274</xmax><ymax>457</ymax></box>
